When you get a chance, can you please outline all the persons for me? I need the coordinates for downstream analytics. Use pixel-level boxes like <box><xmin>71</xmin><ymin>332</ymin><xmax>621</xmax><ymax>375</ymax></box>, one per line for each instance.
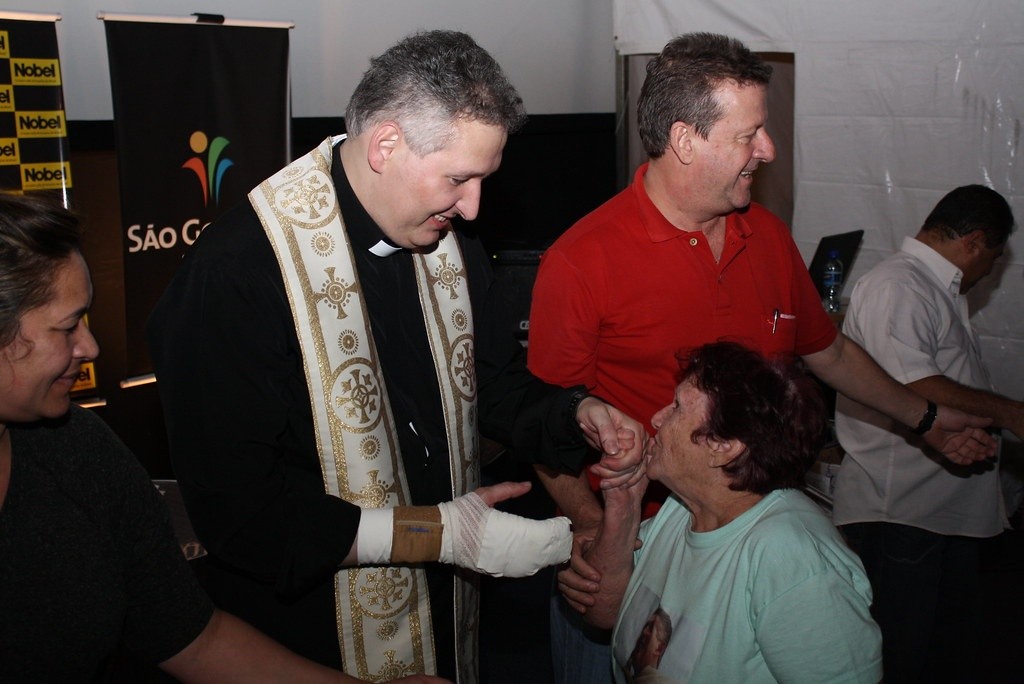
<box><xmin>0</xmin><ymin>194</ymin><xmax>451</xmax><ymax>683</ymax></box>
<box><xmin>204</xmin><ymin>31</ymin><xmax>649</xmax><ymax>684</ymax></box>
<box><xmin>526</xmin><ymin>31</ymin><xmax>997</xmax><ymax>684</ymax></box>
<box><xmin>579</xmin><ymin>341</ymin><xmax>883</xmax><ymax>684</ymax></box>
<box><xmin>833</xmin><ymin>185</ymin><xmax>1024</xmax><ymax>684</ymax></box>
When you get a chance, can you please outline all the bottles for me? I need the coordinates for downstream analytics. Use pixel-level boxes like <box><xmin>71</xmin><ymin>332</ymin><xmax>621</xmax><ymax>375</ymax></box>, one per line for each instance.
<box><xmin>822</xmin><ymin>251</ymin><xmax>843</xmax><ymax>313</ymax></box>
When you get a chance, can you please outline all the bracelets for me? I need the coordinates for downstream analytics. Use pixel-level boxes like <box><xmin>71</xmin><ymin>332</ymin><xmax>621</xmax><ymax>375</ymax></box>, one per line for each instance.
<box><xmin>909</xmin><ymin>400</ymin><xmax>938</xmax><ymax>433</ymax></box>
<box><xmin>571</xmin><ymin>392</ymin><xmax>616</xmax><ymax>436</ymax></box>
